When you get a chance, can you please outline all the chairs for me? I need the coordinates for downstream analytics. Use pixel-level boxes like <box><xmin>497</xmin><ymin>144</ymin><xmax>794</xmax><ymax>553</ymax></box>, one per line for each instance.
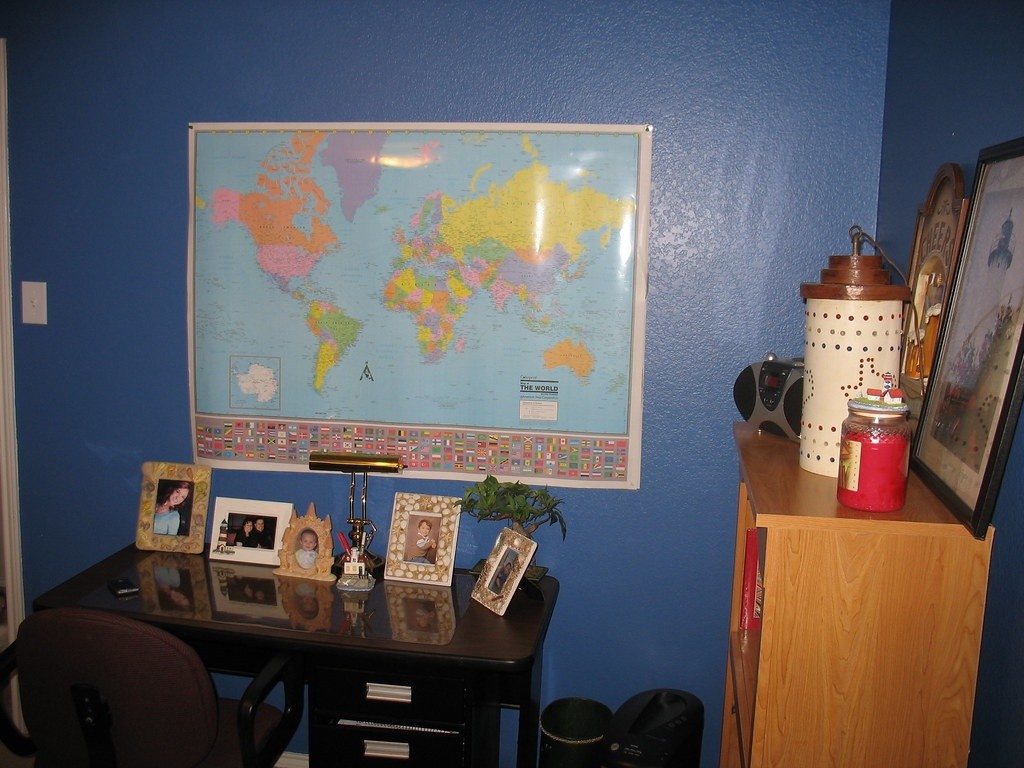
<box><xmin>0</xmin><ymin>608</ymin><xmax>305</xmax><ymax>768</ymax></box>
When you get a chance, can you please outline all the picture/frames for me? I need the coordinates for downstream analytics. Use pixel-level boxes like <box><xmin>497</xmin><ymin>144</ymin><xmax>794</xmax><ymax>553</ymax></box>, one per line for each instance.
<box><xmin>908</xmin><ymin>135</ymin><xmax>1024</xmax><ymax>541</ymax></box>
<box><xmin>209</xmin><ymin>496</ymin><xmax>293</xmax><ymax>566</ymax></box>
<box><xmin>899</xmin><ymin>161</ymin><xmax>970</xmax><ymax>464</ymax></box>
<box><xmin>135</xmin><ymin>460</ymin><xmax>212</xmax><ymax>554</ymax></box>
<box><xmin>137</xmin><ymin>553</ymin><xmax>211</xmax><ymax>622</ymax></box>
<box><xmin>209</xmin><ymin>561</ymin><xmax>290</xmax><ymax>621</ymax></box>
<box><xmin>384</xmin><ymin>581</ymin><xmax>457</xmax><ymax>645</ymax></box>
<box><xmin>273</xmin><ymin>502</ymin><xmax>337</xmax><ymax>581</ymax></box>
<box><xmin>277</xmin><ymin>576</ymin><xmax>334</xmax><ymax>634</ymax></box>
<box><xmin>383</xmin><ymin>491</ymin><xmax>462</xmax><ymax>587</ymax></box>
<box><xmin>470</xmin><ymin>528</ymin><xmax>538</xmax><ymax>618</ymax></box>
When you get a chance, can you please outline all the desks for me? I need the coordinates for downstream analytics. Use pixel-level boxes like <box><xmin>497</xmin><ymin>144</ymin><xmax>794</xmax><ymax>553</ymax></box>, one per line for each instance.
<box><xmin>32</xmin><ymin>540</ymin><xmax>560</xmax><ymax>768</ymax></box>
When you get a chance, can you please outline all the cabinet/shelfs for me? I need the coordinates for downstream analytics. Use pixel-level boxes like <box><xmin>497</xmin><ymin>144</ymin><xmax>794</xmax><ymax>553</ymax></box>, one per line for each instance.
<box><xmin>720</xmin><ymin>421</ymin><xmax>995</xmax><ymax>768</ymax></box>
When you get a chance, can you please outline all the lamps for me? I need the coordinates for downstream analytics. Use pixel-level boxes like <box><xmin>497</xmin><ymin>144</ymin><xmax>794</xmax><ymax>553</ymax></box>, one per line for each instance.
<box><xmin>309</xmin><ymin>452</ymin><xmax>407</xmax><ymax>591</ymax></box>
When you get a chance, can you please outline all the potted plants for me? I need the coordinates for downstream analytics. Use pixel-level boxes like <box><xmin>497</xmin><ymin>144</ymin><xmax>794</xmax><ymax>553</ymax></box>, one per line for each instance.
<box><xmin>453</xmin><ymin>475</ymin><xmax>567</xmax><ymax>588</ymax></box>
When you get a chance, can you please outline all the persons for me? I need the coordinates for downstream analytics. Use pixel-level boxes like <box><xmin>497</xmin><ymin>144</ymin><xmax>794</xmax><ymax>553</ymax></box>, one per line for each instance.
<box><xmin>295</xmin><ymin>529</ymin><xmax>319</xmax><ymax>568</ymax></box>
<box><xmin>410</xmin><ymin>518</ymin><xmax>436</xmax><ymax>563</ymax></box>
<box><xmin>492</xmin><ymin>562</ymin><xmax>512</xmax><ymax>594</ymax></box>
<box><xmin>155</xmin><ymin>565</ymin><xmax>439</xmax><ymax>633</ymax></box>
<box><xmin>153</xmin><ymin>481</ymin><xmax>193</xmax><ymax>536</ymax></box>
<box><xmin>230</xmin><ymin>517</ymin><xmax>274</xmax><ymax>550</ymax></box>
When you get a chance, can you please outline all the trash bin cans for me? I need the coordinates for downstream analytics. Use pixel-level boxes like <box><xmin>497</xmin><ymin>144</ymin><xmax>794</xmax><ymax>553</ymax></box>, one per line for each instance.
<box><xmin>537</xmin><ymin>697</ymin><xmax>613</xmax><ymax>768</ymax></box>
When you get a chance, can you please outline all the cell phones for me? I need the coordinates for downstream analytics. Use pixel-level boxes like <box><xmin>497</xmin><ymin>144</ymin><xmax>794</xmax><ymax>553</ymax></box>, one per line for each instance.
<box><xmin>107</xmin><ymin>577</ymin><xmax>141</xmax><ymax>597</ymax></box>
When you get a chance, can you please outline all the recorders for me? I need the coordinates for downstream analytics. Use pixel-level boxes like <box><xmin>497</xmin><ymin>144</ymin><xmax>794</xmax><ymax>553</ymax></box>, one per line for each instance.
<box><xmin>733</xmin><ymin>352</ymin><xmax>804</xmax><ymax>444</ymax></box>
